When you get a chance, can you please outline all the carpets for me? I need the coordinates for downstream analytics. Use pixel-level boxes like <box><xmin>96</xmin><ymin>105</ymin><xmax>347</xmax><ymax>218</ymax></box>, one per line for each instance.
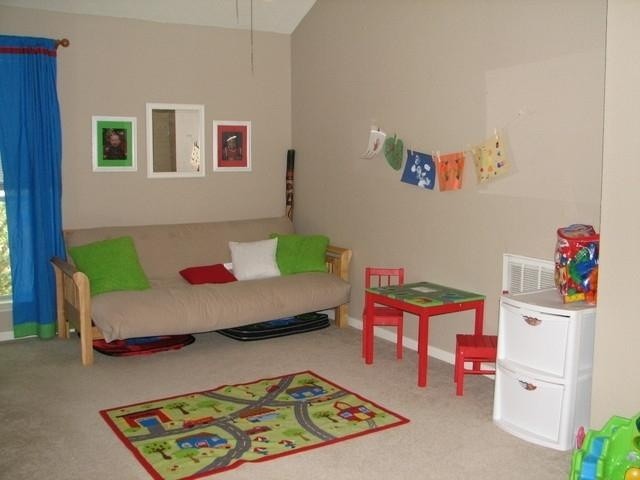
<box><xmin>99</xmin><ymin>369</ymin><xmax>411</xmax><ymax>480</ymax></box>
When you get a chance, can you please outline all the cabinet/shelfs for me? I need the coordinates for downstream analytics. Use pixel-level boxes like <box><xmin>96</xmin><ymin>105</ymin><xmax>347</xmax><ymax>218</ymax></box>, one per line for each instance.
<box><xmin>493</xmin><ymin>286</ymin><xmax>594</xmax><ymax>453</ymax></box>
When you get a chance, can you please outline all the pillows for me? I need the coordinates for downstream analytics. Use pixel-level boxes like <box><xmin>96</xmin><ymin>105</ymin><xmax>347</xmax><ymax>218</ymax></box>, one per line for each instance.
<box><xmin>269</xmin><ymin>231</ymin><xmax>330</xmax><ymax>277</ymax></box>
<box><xmin>177</xmin><ymin>263</ymin><xmax>237</xmax><ymax>287</ymax></box>
<box><xmin>227</xmin><ymin>236</ymin><xmax>280</xmax><ymax>281</ymax></box>
<box><xmin>68</xmin><ymin>234</ymin><xmax>150</xmax><ymax>297</ymax></box>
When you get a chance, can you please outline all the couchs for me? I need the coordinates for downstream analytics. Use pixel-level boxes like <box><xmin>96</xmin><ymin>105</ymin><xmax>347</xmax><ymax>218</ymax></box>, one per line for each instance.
<box><xmin>49</xmin><ymin>213</ymin><xmax>353</xmax><ymax>368</ymax></box>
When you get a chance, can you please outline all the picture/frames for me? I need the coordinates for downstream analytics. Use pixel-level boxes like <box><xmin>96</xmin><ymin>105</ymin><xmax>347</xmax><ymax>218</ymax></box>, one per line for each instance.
<box><xmin>213</xmin><ymin>120</ymin><xmax>252</xmax><ymax>171</ymax></box>
<box><xmin>91</xmin><ymin>113</ymin><xmax>139</xmax><ymax>173</ymax></box>
<box><xmin>146</xmin><ymin>102</ymin><xmax>206</xmax><ymax>178</ymax></box>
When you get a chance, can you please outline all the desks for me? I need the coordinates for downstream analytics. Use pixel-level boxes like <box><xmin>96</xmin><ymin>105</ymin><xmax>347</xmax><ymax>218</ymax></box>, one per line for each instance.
<box><xmin>362</xmin><ymin>281</ymin><xmax>485</xmax><ymax>386</ymax></box>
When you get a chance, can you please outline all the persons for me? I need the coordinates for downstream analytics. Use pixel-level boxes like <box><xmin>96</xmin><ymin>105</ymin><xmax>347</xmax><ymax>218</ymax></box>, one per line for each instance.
<box><xmin>106</xmin><ymin>132</ymin><xmax>126</xmax><ymax>160</ymax></box>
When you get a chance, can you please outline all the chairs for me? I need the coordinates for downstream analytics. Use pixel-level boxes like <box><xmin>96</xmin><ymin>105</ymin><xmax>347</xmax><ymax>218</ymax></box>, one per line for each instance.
<box><xmin>452</xmin><ymin>334</ymin><xmax>497</xmax><ymax>396</ymax></box>
<box><xmin>362</xmin><ymin>266</ymin><xmax>404</xmax><ymax>361</ymax></box>
